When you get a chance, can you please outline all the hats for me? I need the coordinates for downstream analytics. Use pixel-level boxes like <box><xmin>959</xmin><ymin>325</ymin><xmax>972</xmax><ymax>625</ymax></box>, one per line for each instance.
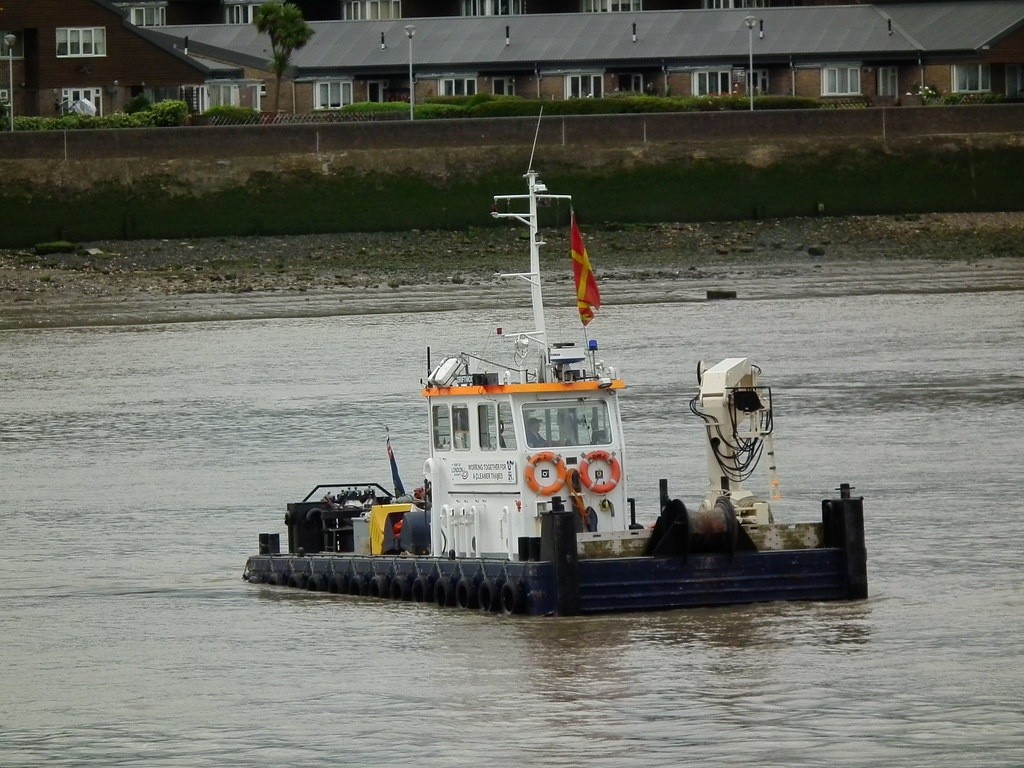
<box><xmin>526</xmin><ymin>418</ymin><xmax>542</xmax><ymax>425</ymax></box>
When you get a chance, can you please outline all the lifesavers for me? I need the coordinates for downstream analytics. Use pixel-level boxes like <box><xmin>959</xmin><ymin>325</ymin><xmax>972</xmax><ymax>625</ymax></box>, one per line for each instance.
<box><xmin>526</xmin><ymin>452</ymin><xmax>567</xmax><ymax>496</ymax></box>
<box><xmin>580</xmin><ymin>451</ymin><xmax>620</xmax><ymax>494</ymax></box>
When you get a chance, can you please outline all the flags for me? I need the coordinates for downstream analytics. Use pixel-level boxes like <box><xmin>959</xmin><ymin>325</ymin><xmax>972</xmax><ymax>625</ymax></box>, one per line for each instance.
<box><xmin>387</xmin><ymin>434</ymin><xmax>406</xmax><ymax>496</ymax></box>
<box><xmin>571</xmin><ymin>211</ymin><xmax>601</xmax><ymax>326</ymax></box>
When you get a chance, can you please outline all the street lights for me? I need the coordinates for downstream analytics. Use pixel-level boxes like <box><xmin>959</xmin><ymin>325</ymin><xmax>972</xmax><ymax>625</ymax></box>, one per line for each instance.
<box><xmin>745</xmin><ymin>15</ymin><xmax>760</xmax><ymax>111</ymax></box>
<box><xmin>4</xmin><ymin>33</ymin><xmax>18</xmax><ymax>133</ymax></box>
<box><xmin>405</xmin><ymin>25</ymin><xmax>418</xmax><ymax>119</ymax></box>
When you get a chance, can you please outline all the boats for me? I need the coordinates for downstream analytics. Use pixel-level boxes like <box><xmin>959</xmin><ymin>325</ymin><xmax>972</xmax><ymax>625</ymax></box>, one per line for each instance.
<box><xmin>244</xmin><ymin>109</ymin><xmax>871</xmax><ymax>622</ymax></box>
